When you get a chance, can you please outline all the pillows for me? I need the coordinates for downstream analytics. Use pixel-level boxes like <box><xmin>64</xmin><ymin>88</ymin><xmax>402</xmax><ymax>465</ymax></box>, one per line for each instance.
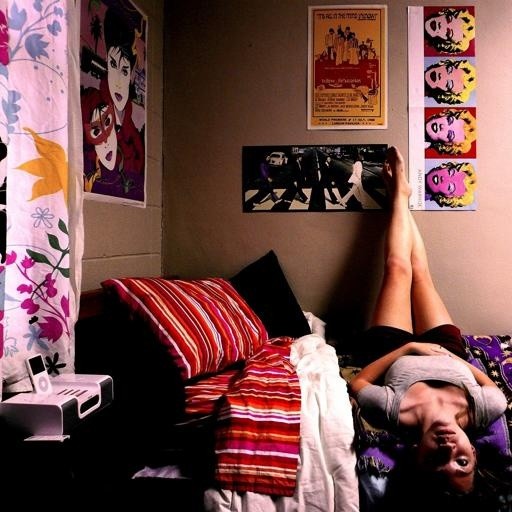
<box><xmin>98</xmin><ymin>274</ymin><xmax>267</xmax><ymax>383</ymax></box>
<box><xmin>230</xmin><ymin>250</ymin><xmax>311</xmax><ymax>339</ymax></box>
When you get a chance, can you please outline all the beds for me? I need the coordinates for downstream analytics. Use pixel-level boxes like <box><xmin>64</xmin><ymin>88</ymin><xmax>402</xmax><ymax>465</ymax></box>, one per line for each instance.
<box><xmin>76</xmin><ymin>274</ymin><xmax>511</xmax><ymax>512</ymax></box>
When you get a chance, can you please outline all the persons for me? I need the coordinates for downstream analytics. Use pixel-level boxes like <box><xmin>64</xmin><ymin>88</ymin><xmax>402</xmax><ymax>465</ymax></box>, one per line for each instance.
<box><xmin>252</xmin><ymin>149</ymin><xmax>368</xmax><ymax>208</ymax></box>
<box><xmin>422</xmin><ymin>4</ymin><xmax>479</xmax><ymax>56</ymax></box>
<box><xmin>425</xmin><ymin>108</ymin><xmax>478</xmax><ymax>156</ymax></box>
<box><xmin>424</xmin><ymin>160</ymin><xmax>479</xmax><ymax>209</ymax></box>
<box><xmin>424</xmin><ymin>57</ymin><xmax>478</xmax><ymax>105</ymax></box>
<box><xmin>77</xmin><ymin>5</ymin><xmax>146</xmax><ymax>201</ymax></box>
<box><xmin>350</xmin><ymin>143</ymin><xmax>508</xmax><ymax>505</ymax></box>
<box><xmin>325</xmin><ymin>22</ymin><xmax>370</xmax><ymax>66</ymax></box>
